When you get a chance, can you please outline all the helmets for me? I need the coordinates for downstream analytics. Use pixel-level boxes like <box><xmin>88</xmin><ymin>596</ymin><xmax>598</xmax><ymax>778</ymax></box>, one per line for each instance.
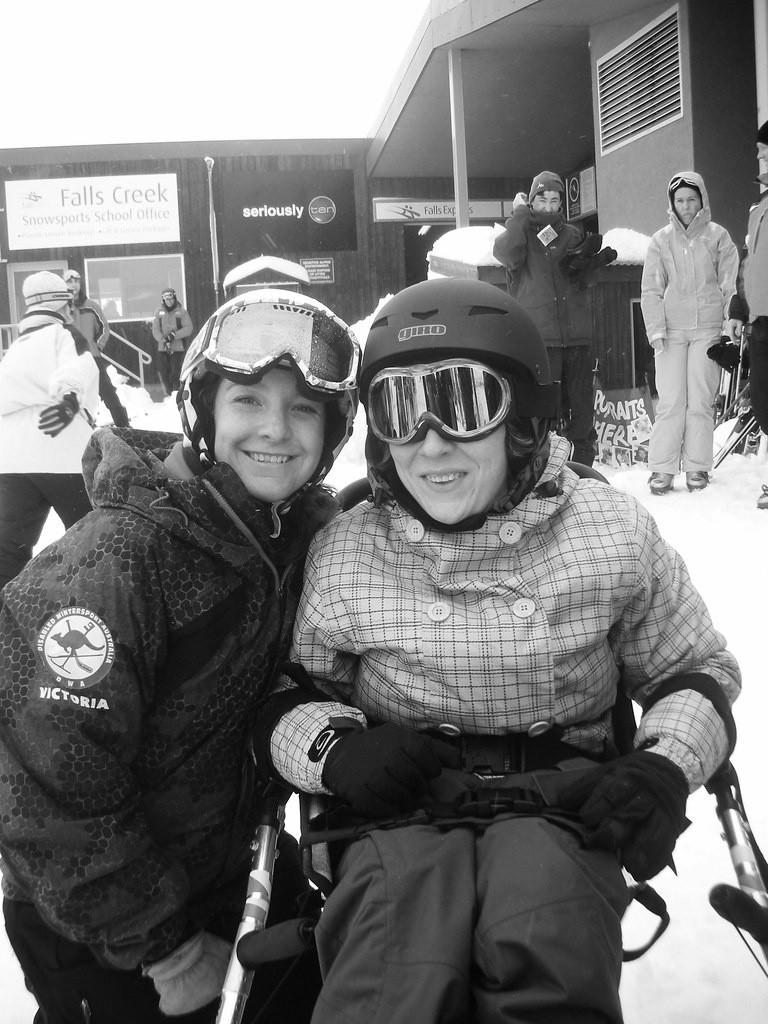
<box><xmin>161</xmin><ymin>288</ymin><xmax>177</xmax><ymax>304</ymax></box>
<box><xmin>356</xmin><ymin>277</ymin><xmax>556</xmax><ymax>435</ymax></box>
<box><xmin>174</xmin><ymin>286</ymin><xmax>363</xmax><ymax>476</ymax></box>
<box><xmin>22</xmin><ymin>270</ymin><xmax>73</xmax><ymax>312</ymax></box>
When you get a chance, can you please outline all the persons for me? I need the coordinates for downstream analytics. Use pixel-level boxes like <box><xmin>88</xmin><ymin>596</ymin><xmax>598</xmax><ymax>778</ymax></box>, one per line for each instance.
<box><xmin>0</xmin><ymin>288</ymin><xmax>364</xmax><ymax>1024</ymax></box>
<box><xmin>151</xmin><ymin>288</ymin><xmax>193</xmax><ymax>396</ymax></box>
<box><xmin>640</xmin><ymin>171</ymin><xmax>740</xmax><ymax>496</ymax></box>
<box><xmin>61</xmin><ymin>269</ymin><xmax>133</xmax><ymax>430</ymax></box>
<box><xmin>725</xmin><ymin>120</ymin><xmax>768</xmax><ymax>511</ymax></box>
<box><xmin>493</xmin><ymin>171</ymin><xmax>618</xmax><ymax>468</ymax></box>
<box><xmin>247</xmin><ymin>276</ymin><xmax>742</xmax><ymax>1024</ymax></box>
<box><xmin>0</xmin><ymin>270</ymin><xmax>99</xmax><ymax>594</ymax></box>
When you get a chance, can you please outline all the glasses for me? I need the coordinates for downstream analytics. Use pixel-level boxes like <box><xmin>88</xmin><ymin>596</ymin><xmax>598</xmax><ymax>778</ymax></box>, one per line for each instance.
<box><xmin>365</xmin><ymin>358</ymin><xmax>562</xmax><ymax>444</ymax></box>
<box><xmin>180</xmin><ymin>294</ymin><xmax>360</xmax><ymax>418</ymax></box>
<box><xmin>161</xmin><ymin>292</ymin><xmax>176</xmax><ymax>301</ymax></box>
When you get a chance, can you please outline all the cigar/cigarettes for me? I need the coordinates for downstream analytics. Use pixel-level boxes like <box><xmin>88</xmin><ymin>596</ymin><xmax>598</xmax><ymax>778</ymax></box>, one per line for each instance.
<box><xmin>726</xmin><ymin>340</ymin><xmax>740</xmax><ymax>345</ymax></box>
<box><xmin>652</xmin><ymin>350</ymin><xmax>664</xmax><ymax>357</ymax></box>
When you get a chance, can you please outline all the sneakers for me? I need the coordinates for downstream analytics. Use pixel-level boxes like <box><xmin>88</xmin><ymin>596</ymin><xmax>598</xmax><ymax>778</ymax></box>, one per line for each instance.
<box><xmin>686</xmin><ymin>472</ymin><xmax>710</xmax><ymax>492</ymax></box>
<box><xmin>647</xmin><ymin>472</ymin><xmax>674</xmax><ymax>495</ymax></box>
<box><xmin>757</xmin><ymin>484</ymin><xmax>768</xmax><ymax>509</ymax></box>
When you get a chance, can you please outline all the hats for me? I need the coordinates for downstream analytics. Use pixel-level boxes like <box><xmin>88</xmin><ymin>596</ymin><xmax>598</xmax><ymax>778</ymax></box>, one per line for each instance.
<box><xmin>669</xmin><ymin>177</ymin><xmax>699</xmax><ymax>193</ymax></box>
<box><xmin>62</xmin><ymin>270</ymin><xmax>81</xmax><ymax>282</ymax></box>
<box><xmin>755</xmin><ymin>119</ymin><xmax>768</xmax><ymax>147</ymax></box>
<box><xmin>529</xmin><ymin>171</ymin><xmax>564</xmax><ymax>206</ymax></box>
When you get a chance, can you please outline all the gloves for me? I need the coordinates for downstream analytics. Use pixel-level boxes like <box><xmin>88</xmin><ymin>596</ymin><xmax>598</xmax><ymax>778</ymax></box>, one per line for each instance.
<box><xmin>162</xmin><ymin>341</ymin><xmax>173</xmax><ymax>355</ymax></box>
<box><xmin>38</xmin><ymin>393</ymin><xmax>80</xmax><ymax>438</ymax></box>
<box><xmin>558</xmin><ymin>232</ymin><xmax>618</xmax><ymax>291</ymax></box>
<box><xmin>321</xmin><ymin>715</ymin><xmax>443</xmax><ymax>821</ymax></box>
<box><xmin>141</xmin><ymin>925</ymin><xmax>235</xmax><ymax>1017</ymax></box>
<box><xmin>707</xmin><ymin>335</ymin><xmax>741</xmax><ymax>374</ymax></box>
<box><xmin>163</xmin><ymin>331</ymin><xmax>174</xmax><ymax>345</ymax></box>
<box><xmin>560</xmin><ymin>751</ymin><xmax>693</xmax><ymax>880</ymax></box>
